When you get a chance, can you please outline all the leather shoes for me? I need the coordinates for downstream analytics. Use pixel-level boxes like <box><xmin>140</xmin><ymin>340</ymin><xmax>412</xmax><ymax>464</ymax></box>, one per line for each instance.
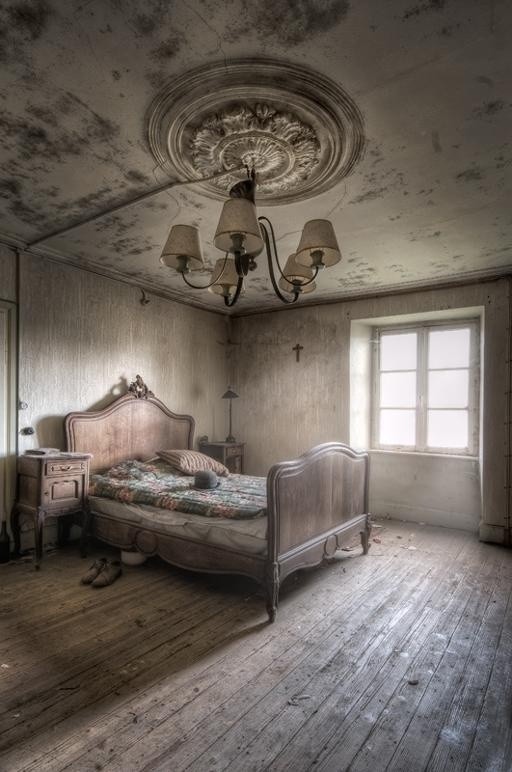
<box><xmin>81</xmin><ymin>557</ymin><xmax>122</xmax><ymax>588</ymax></box>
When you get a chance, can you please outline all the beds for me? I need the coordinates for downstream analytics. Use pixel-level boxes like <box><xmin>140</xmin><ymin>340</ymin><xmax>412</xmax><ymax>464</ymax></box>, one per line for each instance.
<box><xmin>64</xmin><ymin>375</ymin><xmax>372</xmax><ymax>622</ymax></box>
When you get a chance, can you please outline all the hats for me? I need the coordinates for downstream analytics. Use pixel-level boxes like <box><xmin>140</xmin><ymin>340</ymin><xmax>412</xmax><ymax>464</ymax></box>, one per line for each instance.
<box><xmin>190</xmin><ymin>470</ymin><xmax>221</xmax><ymax>492</ymax></box>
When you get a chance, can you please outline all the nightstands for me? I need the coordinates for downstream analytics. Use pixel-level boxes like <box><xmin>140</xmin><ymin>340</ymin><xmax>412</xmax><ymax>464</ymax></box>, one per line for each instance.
<box><xmin>10</xmin><ymin>452</ymin><xmax>92</xmax><ymax>561</ymax></box>
<box><xmin>200</xmin><ymin>443</ymin><xmax>245</xmax><ymax>474</ymax></box>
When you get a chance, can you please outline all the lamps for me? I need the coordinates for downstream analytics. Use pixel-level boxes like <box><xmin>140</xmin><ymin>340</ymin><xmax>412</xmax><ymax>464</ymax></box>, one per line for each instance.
<box><xmin>160</xmin><ymin>166</ymin><xmax>343</xmax><ymax>307</ymax></box>
<box><xmin>221</xmin><ymin>385</ymin><xmax>239</xmax><ymax>443</ymax></box>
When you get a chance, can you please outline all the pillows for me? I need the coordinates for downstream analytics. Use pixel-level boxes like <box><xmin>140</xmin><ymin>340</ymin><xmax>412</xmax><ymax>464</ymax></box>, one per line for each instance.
<box><xmin>156</xmin><ymin>450</ymin><xmax>229</xmax><ymax>478</ymax></box>
